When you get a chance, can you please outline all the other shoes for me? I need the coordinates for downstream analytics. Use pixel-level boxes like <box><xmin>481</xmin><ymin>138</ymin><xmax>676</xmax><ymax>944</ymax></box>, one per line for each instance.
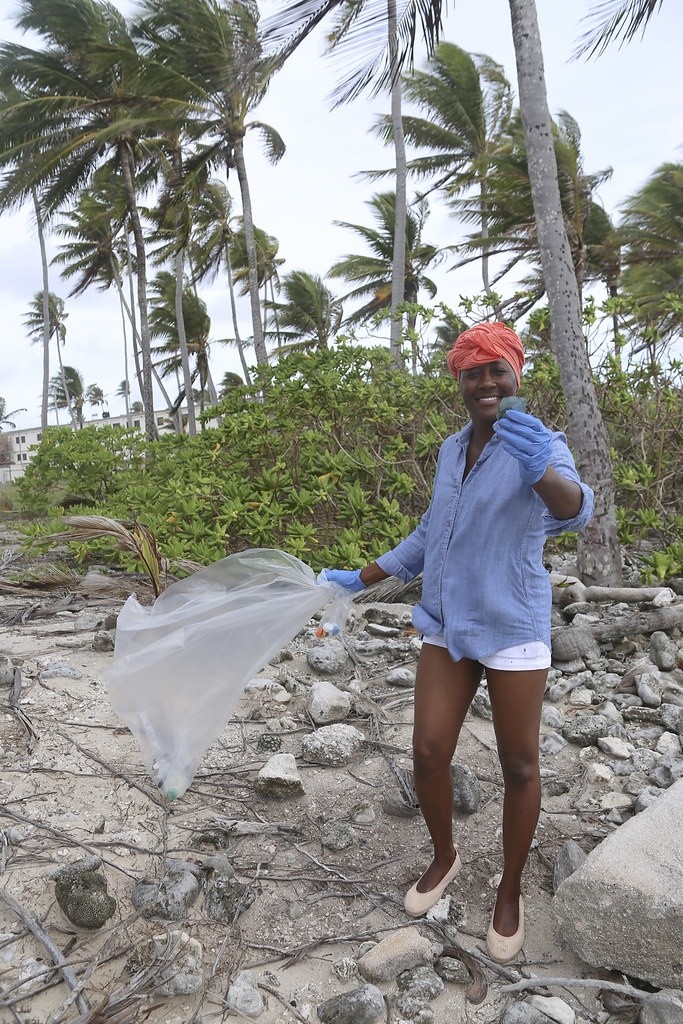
<box><xmin>485</xmin><ymin>893</ymin><xmax>524</xmax><ymax>963</ymax></box>
<box><xmin>404</xmin><ymin>849</ymin><xmax>462</xmax><ymax>916</ymax></box>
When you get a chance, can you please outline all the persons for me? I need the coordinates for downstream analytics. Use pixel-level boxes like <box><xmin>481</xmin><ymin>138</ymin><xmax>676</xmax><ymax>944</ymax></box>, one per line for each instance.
<box><xmin>317</xmin><ymin>322</ymin><xmax>595</xmax><ymax>963</ymax></box>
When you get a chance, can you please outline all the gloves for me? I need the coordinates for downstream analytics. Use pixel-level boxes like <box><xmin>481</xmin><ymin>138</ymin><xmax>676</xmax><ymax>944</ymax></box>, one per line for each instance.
<box><xmin>324</xmin><ymin>568</ymin><xmax>367</xmax><ymax>595</ymax></box>
<box><xmin>494</xmin><ymin>409</ymin><xmax>555</xmax><ymax>484</ymax></box>
